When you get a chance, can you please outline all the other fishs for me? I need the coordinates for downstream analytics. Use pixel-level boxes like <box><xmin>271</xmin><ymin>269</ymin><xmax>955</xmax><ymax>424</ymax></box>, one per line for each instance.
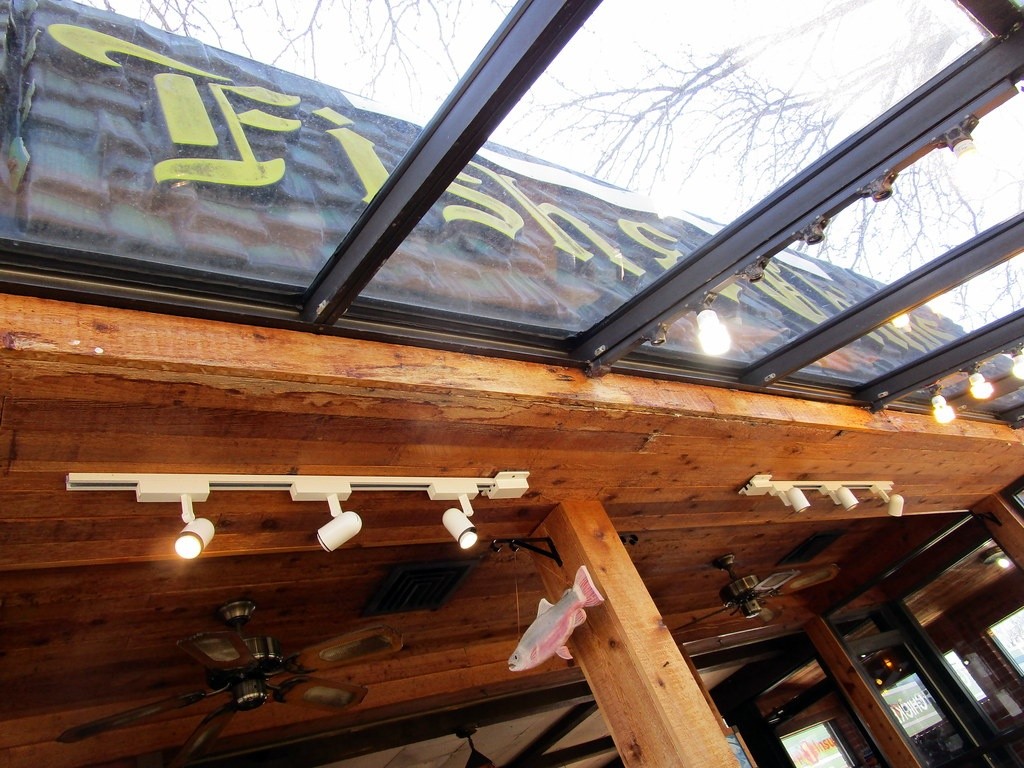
<box><xmin>508</xmin><ymin>565</ymin><xmax>605</xmax><ymax>671</ymax></box>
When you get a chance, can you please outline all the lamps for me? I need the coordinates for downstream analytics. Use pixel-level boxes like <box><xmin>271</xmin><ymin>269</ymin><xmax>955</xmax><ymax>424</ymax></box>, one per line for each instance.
<box><xmin>771</xmin><ymin>484</ymin><xmax>812</xmax><ymax>514</ymax></box>
<box><xmin>134</xmin><ymin>475</ymin><xmax>215</xmax><ymax>560</ymax></box>
<box><xmin>930</xmin><ymin>385</ymin><xmax>958</xmax><ymax>425</ymax></box>
<box><xmin>425</xmin><ymin>480</ymin><xmax>483</xmax><ymax>549</ymax></box>
<box><xmin>869</xmin><ymin>484</ymin><xmax>905</xmax><ymax>516</ymax></box>
<box><xmin>820</xmin><ymin>485</ymin><xmax>861</xmax><ymax>511</ymax></box>
<box><xmin>288</xmin><ymin>478</ymin><xmax>362</xmax><ymax>553</ymax></box>
<box><xmin>685</xmin><ymin>291</ymin><xmax>734</xmax><ymax>357</ymax></box>
<box><xmin>1004</xmin><ymin>347</ymin><xmax>1024</xmax><ymax>380</ymax></box>
<box><xmin>961</xmin><ymin>363</ymin><xmax>994</xmax><ymax>398</ymax></box>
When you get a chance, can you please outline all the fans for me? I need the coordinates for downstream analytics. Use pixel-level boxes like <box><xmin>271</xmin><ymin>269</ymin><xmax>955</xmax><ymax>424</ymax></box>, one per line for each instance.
<box><xmin>676</xmin><ymin>552</ymin><xmax>842</xmax><ymax>631</ymax></box>
<box><xmin>54</xmin><ymin>596</ymin><xmax>406</xmax><ymax>768</ymax></box>
